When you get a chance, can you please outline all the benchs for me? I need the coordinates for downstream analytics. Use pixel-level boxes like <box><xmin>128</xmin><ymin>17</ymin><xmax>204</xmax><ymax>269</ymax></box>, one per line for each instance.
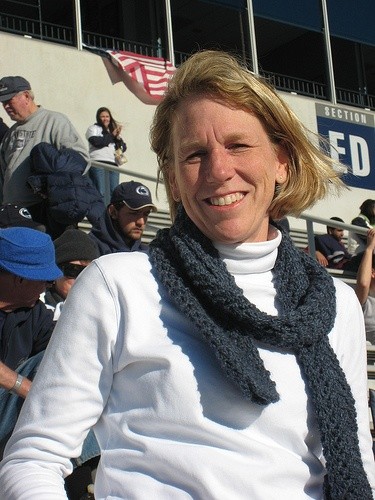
<box><xmin>76</xmin><ymin>206</ymin><xmax>375</xmax><ymax>386</ymax></box>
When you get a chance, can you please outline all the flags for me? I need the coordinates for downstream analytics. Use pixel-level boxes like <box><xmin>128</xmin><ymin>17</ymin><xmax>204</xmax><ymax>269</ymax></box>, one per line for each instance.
<box><xmin>83</xmin><ymin>44</ymin><xmax>177</xmax><ymax>100</ymax></box>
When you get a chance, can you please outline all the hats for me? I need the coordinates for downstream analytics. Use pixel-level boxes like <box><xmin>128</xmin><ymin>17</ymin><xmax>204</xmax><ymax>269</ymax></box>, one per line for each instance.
<box><xmin>111</xmin><ymin>181</ymin><xmax>156</xmax><ymax>213</ymax></box>
<box><xmin>0</xmin><ymin>76</ymin><xmax>31</xmax><ymax>102</ymax></box>
<box><xmin>0</xmin><ymin>201</ymin><xmax>46</xmax><ymax>231</ymax></box>
<box><xmin>53</xmin><ymin>229</ymin><xmax>99</xmax><ymax>264</ymax></box>
<box><xmin>0</xmin><ymin>226</ymin><xmax>63</xmax><ymax>280</ymax></box>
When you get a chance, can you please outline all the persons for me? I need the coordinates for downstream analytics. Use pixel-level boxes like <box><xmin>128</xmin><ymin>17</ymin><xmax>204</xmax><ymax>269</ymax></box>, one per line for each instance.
<box><xmin>0</xmin><ymin>50</ymin><xmax>375</xmax><ymax>500</ymax></box>
<box><xmin>304</xmin><ymin>197</ymin><xmax>375</xmax><ymax>350</ymax></box>
<box><xmin>0</xmin><ymin>75</ymin><xmax>157</xmax><ymax>500</ymax></box>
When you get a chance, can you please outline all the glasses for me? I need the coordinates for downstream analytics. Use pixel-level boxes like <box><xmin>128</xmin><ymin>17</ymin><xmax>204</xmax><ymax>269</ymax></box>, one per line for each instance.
<box><xmin>59</xmin><ymin>262</ymin><xmax>86</xmax><ymax>278</ymax></box>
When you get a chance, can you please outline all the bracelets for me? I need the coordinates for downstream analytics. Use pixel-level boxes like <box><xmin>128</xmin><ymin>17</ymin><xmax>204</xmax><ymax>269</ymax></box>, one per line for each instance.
<box><xmin>7</xmin><ymin>373</ymin><xmax>24</xmax><ymax>394</ymax></box>
